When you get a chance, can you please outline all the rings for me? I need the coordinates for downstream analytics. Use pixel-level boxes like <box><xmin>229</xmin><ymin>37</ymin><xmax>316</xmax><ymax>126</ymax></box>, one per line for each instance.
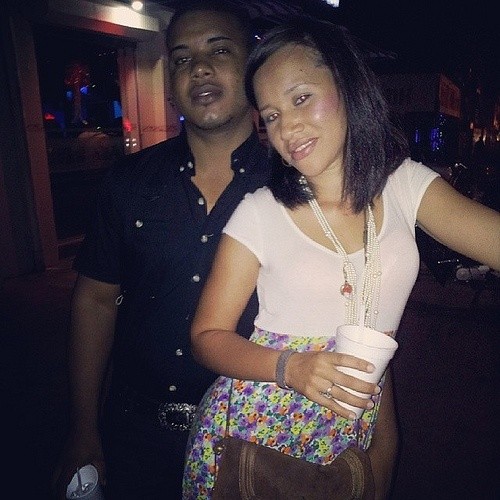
<box><xmin>327</xmin><ymin>382</ymin><xmax>335</xmax><ymax>395</ymax></box>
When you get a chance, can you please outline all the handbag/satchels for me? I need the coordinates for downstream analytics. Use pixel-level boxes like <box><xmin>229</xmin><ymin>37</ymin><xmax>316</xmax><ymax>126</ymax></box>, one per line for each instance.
<box><xmin>211</xmin><ymin>437</ymin><xmax>375</xmax><ymax>499</ymax></box>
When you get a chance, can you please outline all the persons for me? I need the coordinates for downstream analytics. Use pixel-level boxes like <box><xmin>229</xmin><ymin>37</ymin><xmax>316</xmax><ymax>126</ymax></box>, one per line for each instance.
<box><xmin>179</xmin><ymin>18</ymin><xmax>500</xmax><ymax>500</ymax></box>
<box><xmin>66</xmin><ymin>0</ymin><xmax>271</xmax><ymax>500</ymax></box>
<box><xmin>76</xmin><ymin>116</ymin><xmax>112</xmax><ymax>188</ymax></box>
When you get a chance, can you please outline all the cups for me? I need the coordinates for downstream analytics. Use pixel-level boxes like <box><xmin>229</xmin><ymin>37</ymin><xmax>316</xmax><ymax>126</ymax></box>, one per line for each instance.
<box><xmin>67</xmin><ymin>465</ymin><xmax>101</xmax><ymax>500</ymax></box>
<box><xmin>333</xmin><ymin>325</ymin><xmax>398</xmax><ymax>418</ymax></box>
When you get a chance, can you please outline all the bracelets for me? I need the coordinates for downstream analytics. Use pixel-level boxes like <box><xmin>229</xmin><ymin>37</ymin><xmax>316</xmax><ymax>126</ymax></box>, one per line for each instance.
<box><xmin>275</xmin><ymin>349</ymin><xmax>297</xmax><ymax>392</ymax></box>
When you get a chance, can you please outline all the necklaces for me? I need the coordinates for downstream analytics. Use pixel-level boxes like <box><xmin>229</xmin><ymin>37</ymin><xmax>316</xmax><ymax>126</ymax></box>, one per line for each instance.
<box><xmin>299</xmin><ymin>171</ymin><xmax>382</xmax><ymax>330</ymax></box>
